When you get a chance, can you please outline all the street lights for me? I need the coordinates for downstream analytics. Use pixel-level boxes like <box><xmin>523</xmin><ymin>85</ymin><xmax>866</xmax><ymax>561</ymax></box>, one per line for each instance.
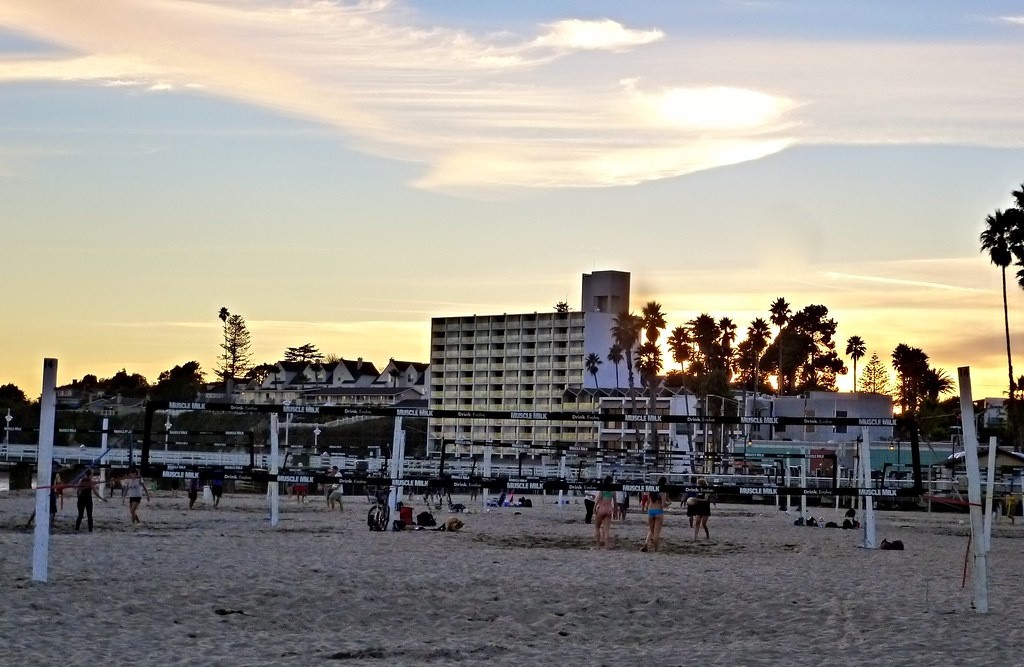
<box><xmin>283</xmin><ymin>398</ymin><xmax>291</xmax><ymax>455</ymax></box>
<box><xmin>889</xmin><ymin>438</ymin><xmax>900</xmax><ymax>488</ymax></box>
<box><xmin>165</xmin><ymin>416</ymin><xmax>173</xmax><ymax>451</ymax></box>
<box><xmin>314</xmin><ymin>423</ymin><xmax>321</xmax><ymax>454</ymax></box>
<box><xmin>744</xmin><ymin>433</ymin><xmax>753</xmax><ymax>477</ymax></box>
<box><xmin>5</xmin><ymin>408</ymin><xmax>14</xmax><ymax>461</ymax></box>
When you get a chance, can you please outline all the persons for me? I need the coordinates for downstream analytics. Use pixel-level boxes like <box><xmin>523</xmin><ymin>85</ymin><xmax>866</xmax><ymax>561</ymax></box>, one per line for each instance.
<box><xmin>73</xmin><ymin>470</ymin><xmax>107</xmax><ymax>533</ymax></box>
<box><xmin>692</xmin><ymin>480</ymin><xmax>712</xmax><ymax>542</ymax></box>
<box><xmin>328</xmin><ymin>466</ymin><xmax>343</xmax><ymax>512</ymax></box>
<box><xmin>210</xmin><ymin>470</ymin><xmax>223</xmax><ymax>506</ymax></box>
<box><xmin>188</xmin><ymin>479</ymin><xmax>198</xmax><ymax>509</ymax></box>
<box><xmin>585</xmin><ymin>487</ymin><xmax>597</xmax><ymax>524</ymax></box>
<box><xmin>469</xmin><ymin>488</ymin><xmax>478</xmax><ymax>501</ymax></box>
<box><xmin>50</xmin><ymin>489</ymin><xmax>56</xmax><ymax>526</ymax></box>
<box><xmin>171</xmin><ymin>478</ymin><xmax>179</xmax><ymax>497</ymax></box>
<box><xmin>641</xmin><ymin>477</ymin><xmax>671</xmax><ymax>553</ymax></box>
<box><xmin>615</xmin><ymin>481</ymin><xmax>626</xmax><ymax>520</ymax></box>
<box><xmin>591</xmin><ymin>477</ymin><xmax>617</xmax><ymax>551</ymax></box>
<box><xmin>110</xmin><ymin>471</ymin><xmax>150</xmax><ymax>522</ymax></box>
<box><xmin>295</xmin><ymin>462</ymin><xmax>308</xmax><ymax>503</ymax></box>
<box><xmin>680</xmin><ymin>478</ymin><xmax>699</xmax><ymax>527</ymax></box>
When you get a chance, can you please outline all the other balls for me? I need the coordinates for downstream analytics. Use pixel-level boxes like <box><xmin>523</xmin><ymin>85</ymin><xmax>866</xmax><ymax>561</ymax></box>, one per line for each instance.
<box><xmin>332</xmin><ymin>484</ymin><xmax>337</xmax><ymax>489</ymax></box>
<box><xmin>687</xmin><ymin>497</ymin><xmax>695</xmax><ymax>505</ymax></box>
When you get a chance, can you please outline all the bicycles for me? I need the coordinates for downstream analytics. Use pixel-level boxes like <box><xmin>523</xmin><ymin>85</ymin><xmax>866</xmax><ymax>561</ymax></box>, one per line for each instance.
<box><xmin>367</xmin><ymin>490</ymin><xmax>392</xmax><ymax>530</ymax></box>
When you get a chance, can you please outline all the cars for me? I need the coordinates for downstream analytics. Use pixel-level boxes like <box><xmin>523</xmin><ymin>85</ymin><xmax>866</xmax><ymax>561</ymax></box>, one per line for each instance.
<box><xmin>2</xmin><ymin>446</ymin><xmax>7</xmax><ymax>452</ymax></box>
